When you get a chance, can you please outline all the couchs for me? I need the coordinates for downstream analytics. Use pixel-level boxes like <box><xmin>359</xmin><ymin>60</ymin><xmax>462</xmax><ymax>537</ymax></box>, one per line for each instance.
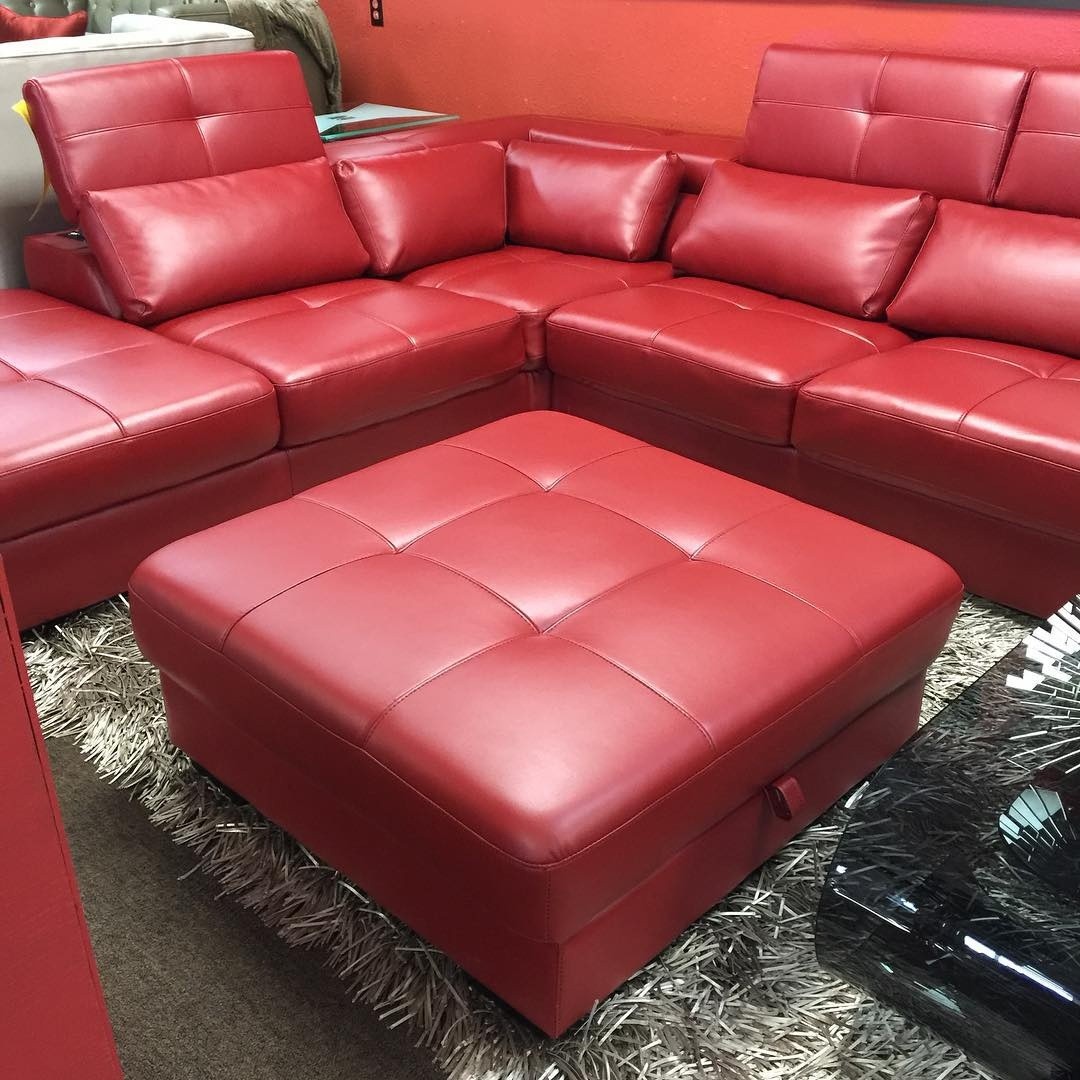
<box><xmin>0</xmin><ymin>0</ymin><xmax>344</xmax><ymax>137</ymax></box>
<box><xmin>1</xmin><ymin>0</ymin><xmax>1080</xmax><ymax>656</ymax></box>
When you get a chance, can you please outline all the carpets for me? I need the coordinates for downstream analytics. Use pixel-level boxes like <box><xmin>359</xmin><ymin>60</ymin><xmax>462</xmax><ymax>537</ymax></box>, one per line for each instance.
<box><xmin>18</xmin><ymin>506</ymin><xmax>1060</xmax><ymax>1080</ymax></box>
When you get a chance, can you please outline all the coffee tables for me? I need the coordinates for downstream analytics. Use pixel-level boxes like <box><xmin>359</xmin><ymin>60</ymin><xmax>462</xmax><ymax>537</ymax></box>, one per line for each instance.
<box><xmin>107</xmin><ymin>408</ymin><xmax>957</xmax><ymax>1039</ymax></box>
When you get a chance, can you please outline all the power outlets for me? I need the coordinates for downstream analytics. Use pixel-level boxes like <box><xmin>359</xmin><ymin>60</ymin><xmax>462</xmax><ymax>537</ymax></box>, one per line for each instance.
<box><xmin>370</xmin><ymin>0</ymin><xmax>385</xmax><ymax>26</ymax></box>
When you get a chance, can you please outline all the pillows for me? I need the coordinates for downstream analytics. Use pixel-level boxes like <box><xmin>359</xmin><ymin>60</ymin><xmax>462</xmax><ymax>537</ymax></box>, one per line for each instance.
<box><xmin>0</xmin><ymin>2</ymin><xmax>91</xmax><ymax>43</ymax></box>
